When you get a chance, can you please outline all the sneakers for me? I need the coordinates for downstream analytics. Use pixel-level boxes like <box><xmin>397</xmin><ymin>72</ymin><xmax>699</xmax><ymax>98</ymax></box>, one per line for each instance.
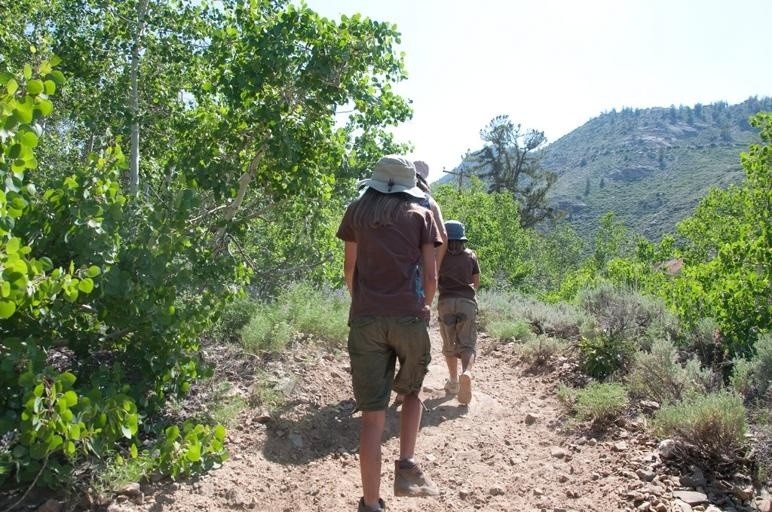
<box><xmin>394</xmin><ymin>459</ymin><xmax>439</xmax><ymax>495</ymax></box>
<box><xmin>444</xmin><ymin>373</ymin><xmax>472</xmax><ymax>402</ymax></box>
<box><xmin>358</xmin><ymin>496</ymin><xmax>386</xmax><ymax>512</ymax></box>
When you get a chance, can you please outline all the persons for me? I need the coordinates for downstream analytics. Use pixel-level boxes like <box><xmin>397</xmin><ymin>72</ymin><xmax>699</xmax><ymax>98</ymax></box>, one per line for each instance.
<box><xmin>437</xmin><ymin>218</ymin><xmax>482</xmax><ymax>407</ymax></box>
<box><xmin>333</xmin><ymin>153</ymin><xmax>441</xmax><ymax>512</ymax></box>
<box><xmin>409</xmin><ymin>159</ymin><xmax>445</xmax><ymax>327</ymax></box>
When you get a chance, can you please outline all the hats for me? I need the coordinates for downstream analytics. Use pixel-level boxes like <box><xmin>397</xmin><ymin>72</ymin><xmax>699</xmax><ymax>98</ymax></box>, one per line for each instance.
<box><xmin>355</xmin><ymin>154</ymin><xmax>429</xmax><ymax>198</ymax></box>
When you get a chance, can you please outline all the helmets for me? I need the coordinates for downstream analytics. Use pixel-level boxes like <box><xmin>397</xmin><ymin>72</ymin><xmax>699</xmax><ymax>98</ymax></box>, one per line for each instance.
<box><xmin>444</xmin><ymin>220</ymin><xmax>467</xmax><ymax>240</ymax></box>
